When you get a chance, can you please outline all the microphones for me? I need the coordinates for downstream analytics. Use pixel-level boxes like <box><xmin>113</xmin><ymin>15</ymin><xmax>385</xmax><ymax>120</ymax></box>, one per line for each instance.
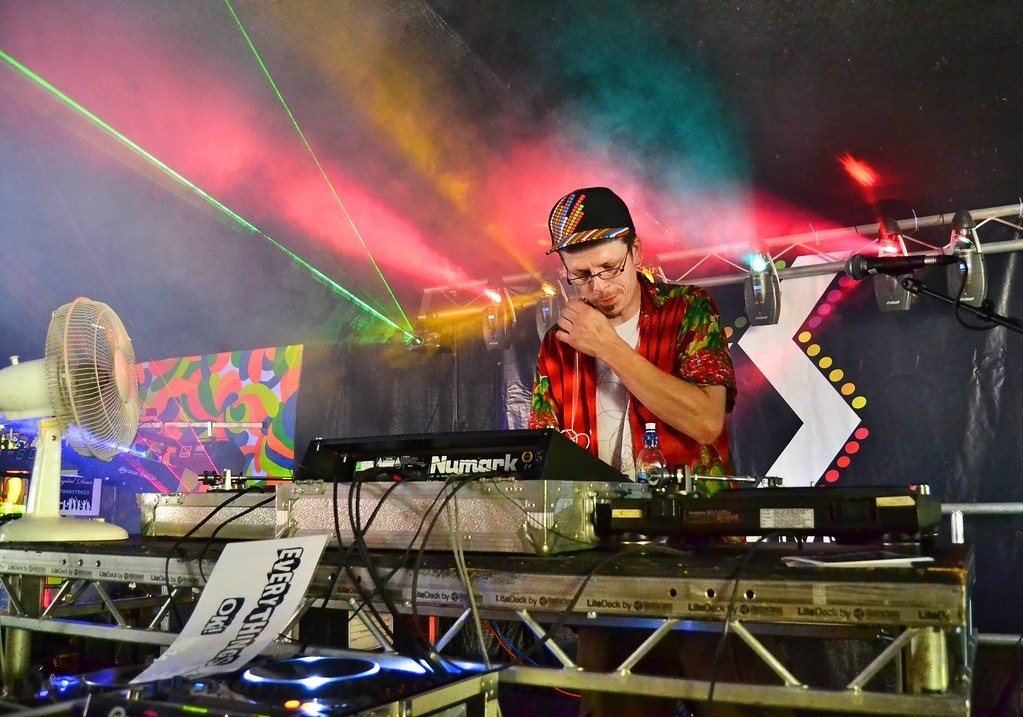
<box><xmin>845</xmin><ymin>255</ymin><xmax>958</xmax><ymax>281</ymax></box>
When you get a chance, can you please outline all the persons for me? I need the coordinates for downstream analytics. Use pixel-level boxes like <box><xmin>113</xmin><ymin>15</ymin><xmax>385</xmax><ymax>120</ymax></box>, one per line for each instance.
<box><xmin>529</xmin><ymin>184</ymin><xmax>749</xmax><ymax>715</ymax></box>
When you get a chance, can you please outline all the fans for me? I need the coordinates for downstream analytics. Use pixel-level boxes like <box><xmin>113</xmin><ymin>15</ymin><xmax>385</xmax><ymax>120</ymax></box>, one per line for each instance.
<box><xmin>0</xmin><ymin>296</ymin><xmax>142</xmax><ymax>544</ymax></box>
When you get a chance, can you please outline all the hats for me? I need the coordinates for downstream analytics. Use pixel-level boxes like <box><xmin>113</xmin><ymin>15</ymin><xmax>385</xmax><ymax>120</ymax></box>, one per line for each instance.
<box><xmin>546</xmin><ymin>187</ymin><xmax>634</xmax><ymax>255</ymax></box>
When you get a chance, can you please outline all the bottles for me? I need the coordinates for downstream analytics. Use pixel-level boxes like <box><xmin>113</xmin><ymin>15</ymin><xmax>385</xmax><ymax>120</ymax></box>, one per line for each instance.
<box><xmin>636</xmin><ymin>421</ymin><xmax>667</xmax><ymax>483</ymax></box>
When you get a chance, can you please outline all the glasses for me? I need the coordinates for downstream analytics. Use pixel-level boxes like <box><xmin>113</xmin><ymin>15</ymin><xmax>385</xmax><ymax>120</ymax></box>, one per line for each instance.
<box><xmin>566</xmin><ymin>244</ymin><xmax>631</xmax><ymax>286</ymax></box>
